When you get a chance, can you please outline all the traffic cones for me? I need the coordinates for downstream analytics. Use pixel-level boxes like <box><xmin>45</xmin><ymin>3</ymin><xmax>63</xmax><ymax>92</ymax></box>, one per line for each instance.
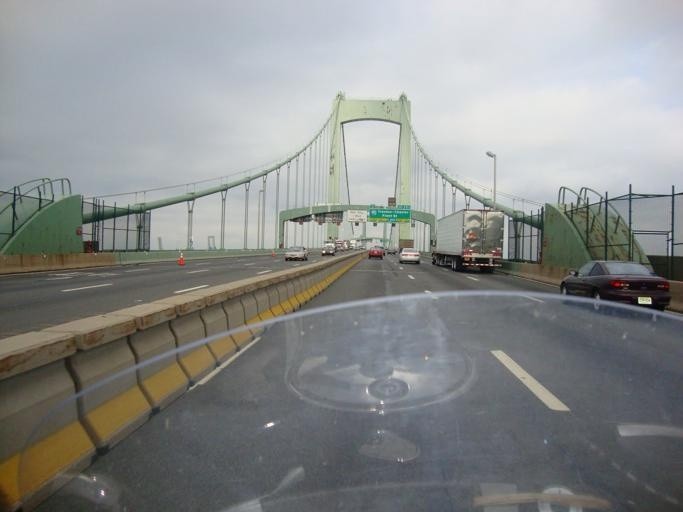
<box><xmin>176</xmin><ymin>252</ymin><xmax>187</xmax><ymax>265</ymax></box>
<box><xmin>271</xmin><ymin>248</ymin><xmax>276</xmax><ymax>257</ymax></box>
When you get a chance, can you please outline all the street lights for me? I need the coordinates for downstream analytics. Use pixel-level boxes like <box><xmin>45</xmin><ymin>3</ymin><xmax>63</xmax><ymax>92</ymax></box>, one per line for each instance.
<box><xmin>485</xmin><ymin>149</ymin><xmax>500</xmax><ymax>210</ymax></box>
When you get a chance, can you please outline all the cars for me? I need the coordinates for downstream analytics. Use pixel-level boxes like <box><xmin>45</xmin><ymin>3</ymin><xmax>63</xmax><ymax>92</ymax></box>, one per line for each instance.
<box><xmin>396</xmin><ymin>248</ymin><xmax>422</xmax><ymax>263</ymax></box>
<box><xmin>366</xmin><ymin>242</ymin><xmax>399</xmax><ymax>259</ymax></box>
<box><xmin>283</xmin><ymin>246</ymin><xmax>310</xmax><ymax>262</ymax></box>
<box><xmin>558</xmin><ymin>258</ymin><xmax>673</xmax><ymax>315</ymax></box>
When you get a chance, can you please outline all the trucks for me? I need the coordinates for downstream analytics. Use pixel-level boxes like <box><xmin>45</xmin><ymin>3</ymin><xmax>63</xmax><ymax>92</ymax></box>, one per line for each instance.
<box><xmin>430</xmin><ymin>208</ymin><xmax>506</xmax><ymax>273</ymax></box>
<box><xmin>320</xmin><ymin>235</ymin><xmax>365</xmax><ymax>256</ymax></box>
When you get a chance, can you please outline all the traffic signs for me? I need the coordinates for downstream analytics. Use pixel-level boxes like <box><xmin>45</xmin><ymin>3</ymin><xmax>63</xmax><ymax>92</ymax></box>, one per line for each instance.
<box><xmin>367</xmin><ymin>207</ymin><xmax>411</xmax><ymax>223</ymax></box>
<box><xmin>345</xmin><ymin>210</ymin><xmax>369</xmax><ymax>223</ymax></box>
<box><xmin>397</xmin><ymin>204</ymin><xmax>411</xmax><ymax>210</ymax></box>
